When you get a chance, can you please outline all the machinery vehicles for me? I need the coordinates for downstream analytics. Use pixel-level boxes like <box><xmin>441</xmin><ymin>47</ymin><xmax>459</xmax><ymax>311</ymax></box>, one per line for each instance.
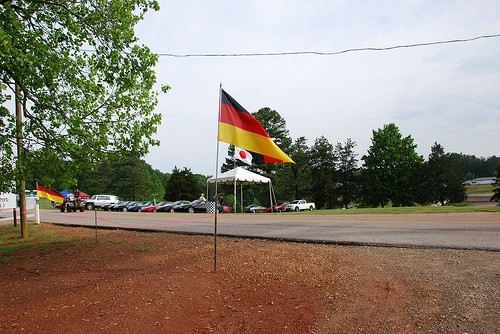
<box><xmin>55</xmin><ymin>186</ymin><xmax>84</xmax><ymax>212</ymax></box>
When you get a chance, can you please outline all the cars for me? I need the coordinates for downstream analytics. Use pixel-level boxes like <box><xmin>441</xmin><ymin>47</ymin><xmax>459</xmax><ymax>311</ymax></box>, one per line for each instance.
<box><xmin>141</xmin><ymin>201</ymin><xmax>171</xmax><ymax>212</ymax></box>
<box><xmin>243</xmin><ymin>204</ymin><xmax>267</xmax><ymax>214</ymax></box>
<box><xmin>156</xmin><ymin>200</ymin><xmax>190</xmax><ymax>212</ymax></box>
<box><xmin>172</xmin><ymin>199</ymin><xmax>213</xmax><ymax>213</ymax></box>
<box><xmin>101</xmin><ymin>201</ymin><xmax>149</xmax><ymax>212</ymax></box>
<box><xmin>268</xmin><ymin>201</ymin><xmax>290</xmax><ymax>212</ymax></box>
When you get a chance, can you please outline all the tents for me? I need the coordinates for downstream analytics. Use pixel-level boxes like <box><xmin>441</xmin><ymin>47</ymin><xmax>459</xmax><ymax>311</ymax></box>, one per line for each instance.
<box><xmin>207</xmin><ymin>168</ymin><xmax>278</xmax><ymax>213</ymax></box>
<box><xmin>60</xmin><ymin>190</ymin><xmax>89</xmax><ymax>198</ymax></box>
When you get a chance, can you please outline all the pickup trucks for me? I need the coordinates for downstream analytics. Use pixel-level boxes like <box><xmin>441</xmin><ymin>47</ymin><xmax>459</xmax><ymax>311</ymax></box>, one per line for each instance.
<box><xmin>283</xmin><ymin>200</ymin><xmax>315</xmax><ymax>211</ymax></box>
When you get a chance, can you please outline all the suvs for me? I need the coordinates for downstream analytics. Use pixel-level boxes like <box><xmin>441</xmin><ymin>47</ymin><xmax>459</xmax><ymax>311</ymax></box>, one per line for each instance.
<box><xmin>83</xmin><ymin>194</ymin><xmax>118</xmax><ymax>210</ymax></box>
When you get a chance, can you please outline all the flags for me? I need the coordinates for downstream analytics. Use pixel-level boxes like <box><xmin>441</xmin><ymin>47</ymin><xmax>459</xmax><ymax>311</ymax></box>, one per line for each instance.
<box><xmin>218</xmin><ymin>90</ymin><xmax>296</xmax><ymax>165</ymax></box>
<box><xmin>37</xmin><ymin>183</ymin><xmax>64</xmax><ymax>203</ymax></box>
<box><xmin>235</xmin><ymin>147</ymin><xmax>253</xmax><ymax>166</ymax></box>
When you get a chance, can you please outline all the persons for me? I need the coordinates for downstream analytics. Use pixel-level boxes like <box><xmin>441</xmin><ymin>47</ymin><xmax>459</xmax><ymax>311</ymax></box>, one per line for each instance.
<box><xmin>212</xmin><ymin>193</ymin><xmax>215</xmax><ymax>202</ymax></box>
<box><xmin>220</xmin><ymin>196</ymin><xmax>224</xmax><ymax>213</ymax></box>
<box><xmin>199</xmin><ymin>194</ymin><xmax>206</xmax><ymax>204</ymax></box>
